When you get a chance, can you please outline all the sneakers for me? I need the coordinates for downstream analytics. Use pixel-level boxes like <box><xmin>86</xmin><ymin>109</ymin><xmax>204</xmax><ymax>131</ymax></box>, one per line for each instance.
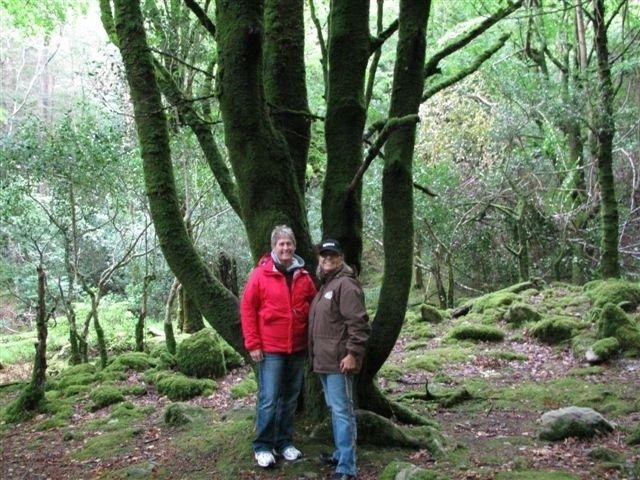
<box><xmin>254</xmin><ymin>451</ymin><xmax>276</xmax><ymax>467</ymax></box>
<box><xmin>329</xmin><ymin>472</ymin><xmax>355</xmax><ymax>480</ymax></box>
<box><xmin>319</xmin><ymin>453</ymin><xmax>337</xmax><ymax>464</ymax></box>
<box><xmin>273</xmin><ymin>444</ymin><xmax>302</xmax><ymax>460</ymax></box>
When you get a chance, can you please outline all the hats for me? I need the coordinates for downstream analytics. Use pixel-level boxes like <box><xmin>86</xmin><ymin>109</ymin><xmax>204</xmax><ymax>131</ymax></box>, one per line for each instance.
<box><xmin>319</xmin><ymin>238</ymin><xmax>342</xmax><ymax>255</ymax></box>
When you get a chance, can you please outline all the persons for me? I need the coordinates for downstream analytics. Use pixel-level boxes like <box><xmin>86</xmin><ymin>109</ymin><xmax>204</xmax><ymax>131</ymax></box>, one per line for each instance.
<box><xmin>308</xmin><ymin>239</ymin><xmax>371</xmax><ymax>480</ymax></box>
<box><xmin>238</xmin><ymin>224</ymin><xmax>318</xmax><ymax>468</ymax></box>
